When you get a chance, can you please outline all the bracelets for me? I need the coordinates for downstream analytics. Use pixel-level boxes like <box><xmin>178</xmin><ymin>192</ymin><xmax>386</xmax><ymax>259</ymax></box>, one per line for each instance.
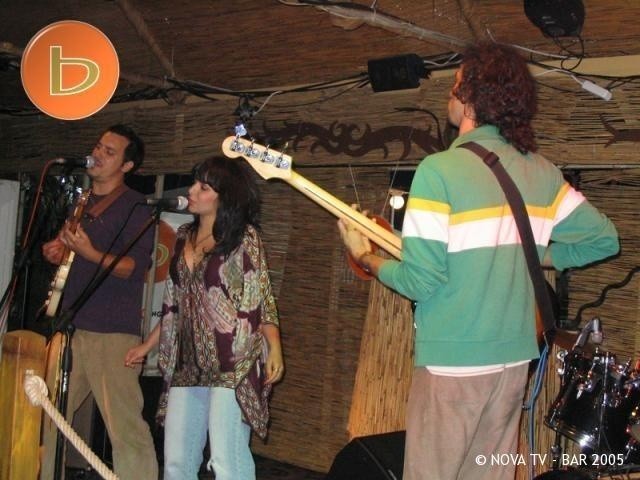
<box><xmin>356</xmin><ymin>250</ymin><xmax>375</xmax><ymax>273</ymax></box>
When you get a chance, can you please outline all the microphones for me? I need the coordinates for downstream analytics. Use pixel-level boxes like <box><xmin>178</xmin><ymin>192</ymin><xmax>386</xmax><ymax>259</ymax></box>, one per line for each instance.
<box><xmin>57</xmin><ymin>154</ymin><xmax>95</xmax><ymax>169</ymax></box>
<box><xmin>591</xmin><ymin>317</ymin><xmax>602</xmax><ymax>344</ymax></box>
<box><xmin>145</xmin><ymin>195</ymin><xmax>190</xmax><ymax>211</ymax></box>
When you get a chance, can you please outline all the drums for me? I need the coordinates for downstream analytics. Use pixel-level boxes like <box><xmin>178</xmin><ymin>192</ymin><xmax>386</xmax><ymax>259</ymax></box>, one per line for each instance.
<box><xmin>545</xmin><ymin>354</ymin><xmax>639</xmax><ymax>455</ymax></box>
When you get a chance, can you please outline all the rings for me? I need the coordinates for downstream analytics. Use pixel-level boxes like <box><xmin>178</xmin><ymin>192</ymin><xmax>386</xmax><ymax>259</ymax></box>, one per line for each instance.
<box><xmin>272</xmin><ymin>366</ymin><xmax>279</xmax><ymax>373</ymax></box>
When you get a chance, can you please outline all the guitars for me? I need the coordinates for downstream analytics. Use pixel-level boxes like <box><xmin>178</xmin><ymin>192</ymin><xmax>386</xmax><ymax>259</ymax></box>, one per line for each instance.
<box><xmin>35</xmin><ymin>190</ymin><xmax>91</xmax><ymax>320</ymax></box>
<box><xmin>221</xmin><ymin>124</ymin><xmax>402</xmax><ymax>260</ymax></box>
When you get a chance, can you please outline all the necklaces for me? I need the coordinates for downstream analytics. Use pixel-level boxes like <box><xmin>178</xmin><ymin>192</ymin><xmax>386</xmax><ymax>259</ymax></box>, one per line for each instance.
<box><xmin>187</xmin><ymin>232</ymin><xmax>212</xmax><ymax>256</ymax></box>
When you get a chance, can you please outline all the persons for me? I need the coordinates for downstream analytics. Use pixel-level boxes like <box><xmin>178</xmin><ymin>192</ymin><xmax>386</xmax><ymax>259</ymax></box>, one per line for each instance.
<box><xmin>39</xmin><ymin>123</ymin><xmax>160</xmax><ymax>480</ymax></box>
<box><xmin>121</xmin><ymin>151</ymin><xmax>286</xmax><ymax>479</ymax></box>
<box><xmin>336</xmin><ymin>38</ymin><xmax>621</xmax><ymax>480</ymax></box>
